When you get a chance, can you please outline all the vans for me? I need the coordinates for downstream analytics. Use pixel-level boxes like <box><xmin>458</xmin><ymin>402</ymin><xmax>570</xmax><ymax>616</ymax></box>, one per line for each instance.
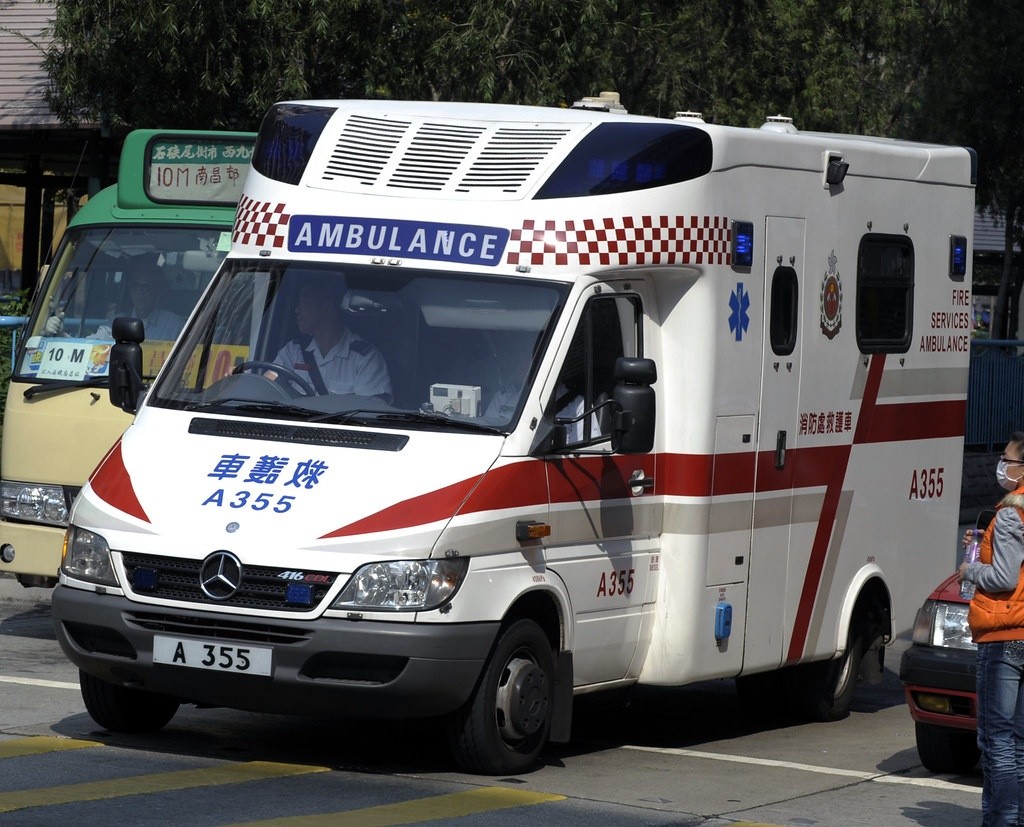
<box><xmin>1</xmin><ymin>126</ymin><xmax>267</xmax><ymax>591</ymax></box>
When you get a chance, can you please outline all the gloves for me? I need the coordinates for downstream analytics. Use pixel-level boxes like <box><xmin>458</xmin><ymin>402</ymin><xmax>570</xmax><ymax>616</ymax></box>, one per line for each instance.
<box><xmin>46</xmin><ymin>311</ymin><xmax>64</xmax><ymax>333</ymax></box>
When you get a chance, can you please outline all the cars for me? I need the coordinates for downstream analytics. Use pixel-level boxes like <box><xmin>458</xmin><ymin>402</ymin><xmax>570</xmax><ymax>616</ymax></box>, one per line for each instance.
<box><xmin>901</xmin><ymin>561</ymin><xmax>983</xmax><ymax>776</ymax></box>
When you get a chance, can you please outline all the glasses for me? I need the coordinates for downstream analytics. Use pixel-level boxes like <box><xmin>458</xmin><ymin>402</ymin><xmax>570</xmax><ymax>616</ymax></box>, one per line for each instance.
<box><xmin>1000</xmin><ymin>455</ymin><xmax>1024</xmax><ymax>464</ymax></box>
<box><xmin>129</xmin><ymin>280</ymin><xmax>152</xmax><ymax>293</ymax></box>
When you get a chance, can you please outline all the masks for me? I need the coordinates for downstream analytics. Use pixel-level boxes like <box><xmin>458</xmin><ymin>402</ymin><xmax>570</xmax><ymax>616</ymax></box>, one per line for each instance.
<box><xmin>996</xmin><ymin>459</ymin><xmax>1024</xmax><ymax>491</ymax></box>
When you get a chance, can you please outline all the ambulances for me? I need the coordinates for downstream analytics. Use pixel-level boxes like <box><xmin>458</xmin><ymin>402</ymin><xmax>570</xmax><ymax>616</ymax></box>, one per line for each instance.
<box><xmin>43</xmin><ymin>86</ymin><xmax>979</xmax><ymax>778</ymax></box>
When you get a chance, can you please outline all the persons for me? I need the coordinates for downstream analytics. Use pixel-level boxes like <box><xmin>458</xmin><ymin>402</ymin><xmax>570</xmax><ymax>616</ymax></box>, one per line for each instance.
<box><xmin>485</xmin><ymin>338</ymin><xmax>602</xmax><ymax>445</ymax></box>
<box><xmin>45</xmin><ymin>263</ymin><xmax>187</xmax><ymax>341</ymax></box>
<box><xmin>956</xmin><ymin>435</ymin><xmax>1024</xmax><ymax>827</ymax></box>
<box><xmin>224</xmin><ymin>277</ymin><xmax>393</xmax><ymax>404</ymax></box>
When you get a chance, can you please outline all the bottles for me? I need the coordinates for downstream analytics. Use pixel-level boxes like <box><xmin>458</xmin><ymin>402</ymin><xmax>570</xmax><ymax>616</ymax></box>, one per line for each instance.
<box><xmin>959</xmin><ymin>528</ymin><xmax>981</xmax><ymax>600</ymax></box>
